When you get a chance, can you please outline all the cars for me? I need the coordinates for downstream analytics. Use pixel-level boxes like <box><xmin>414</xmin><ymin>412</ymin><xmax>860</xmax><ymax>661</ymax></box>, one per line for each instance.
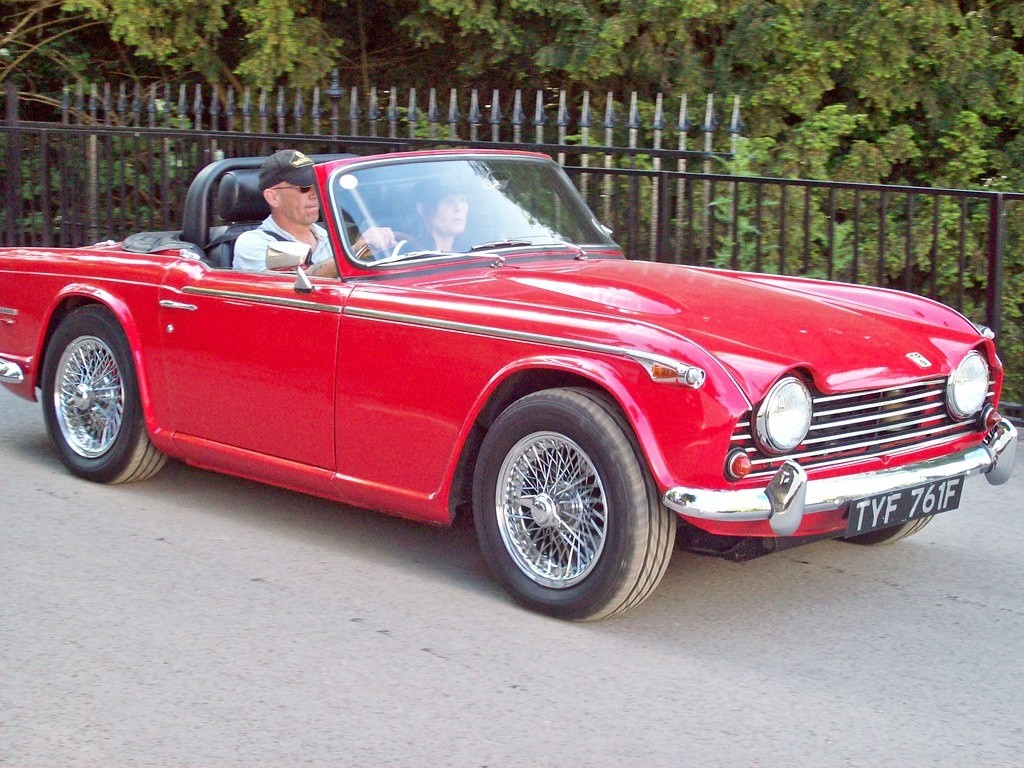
<box><xmin>0</xmin><ymin>147</ymin><xmax>1016</xmax><ymax>622</ymax></box>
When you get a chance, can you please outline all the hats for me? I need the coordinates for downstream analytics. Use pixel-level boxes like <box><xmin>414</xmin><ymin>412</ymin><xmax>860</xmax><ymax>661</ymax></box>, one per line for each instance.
<box><xmin>258</xmin><ymin>149</ymin><xmax>319</xmax><ymax>191</ymax></box>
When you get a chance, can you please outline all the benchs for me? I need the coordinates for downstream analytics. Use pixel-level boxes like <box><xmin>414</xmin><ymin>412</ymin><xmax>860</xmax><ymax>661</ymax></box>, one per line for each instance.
<box><xmin>207</xmin><ymin>164</ymin><xmax>383</xmax><ymax>270</ymax></box>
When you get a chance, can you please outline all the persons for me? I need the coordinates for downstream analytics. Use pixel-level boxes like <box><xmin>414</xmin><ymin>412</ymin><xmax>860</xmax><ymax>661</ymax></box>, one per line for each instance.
<box><xmin>397</xmin><ymin>175</ymin><xmax>497</xmax><ymax>255</ymax></box>
<box><xmin>231</xmin><ymin>149</ymin><xmax>396</xmax><ymax>279</ymax></box>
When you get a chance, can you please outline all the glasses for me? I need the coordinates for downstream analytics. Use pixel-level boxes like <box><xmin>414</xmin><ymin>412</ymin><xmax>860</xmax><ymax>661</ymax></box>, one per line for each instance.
<box><xmin>273</xmin><ymin>184</ymin><xmax>315</xmax><ymax>193</ymax></box>
